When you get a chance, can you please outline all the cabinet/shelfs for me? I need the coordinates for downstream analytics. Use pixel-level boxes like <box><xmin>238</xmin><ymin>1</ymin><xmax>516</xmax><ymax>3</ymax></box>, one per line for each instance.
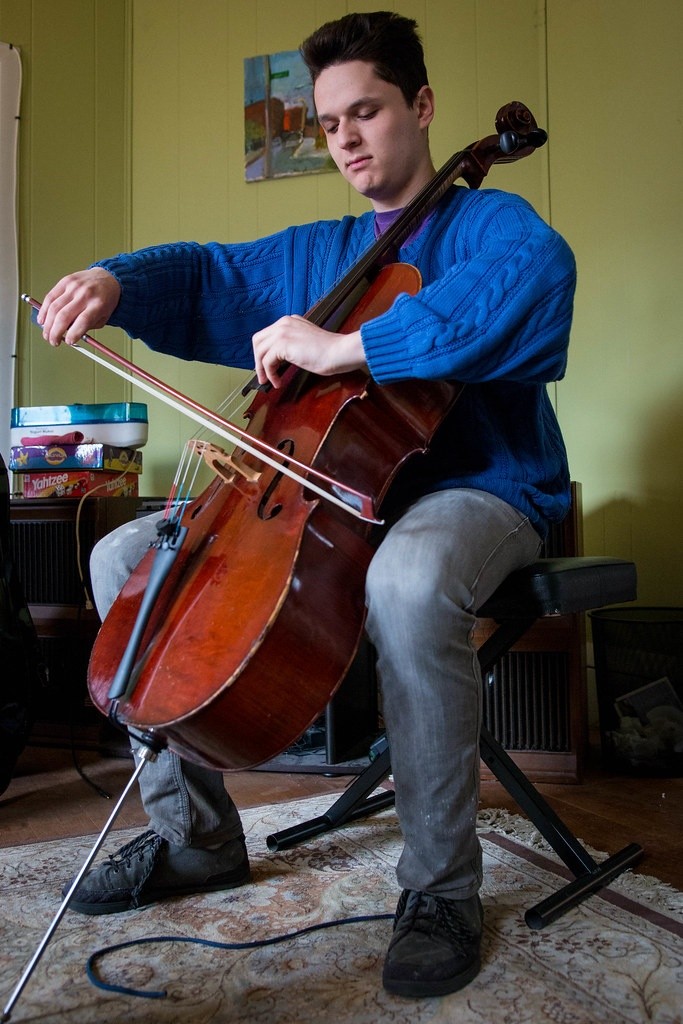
<box><xmin>9</xmin><ymin>483</ymin><xmax>591</xmax><ymax>785</ymax></box>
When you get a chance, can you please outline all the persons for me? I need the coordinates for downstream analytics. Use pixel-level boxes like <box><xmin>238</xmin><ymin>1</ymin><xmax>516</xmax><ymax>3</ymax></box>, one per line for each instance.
<box><xmin>36</xmin><ymin>13</ymin><xmax>577</xmax><ymax>999</ymax></box>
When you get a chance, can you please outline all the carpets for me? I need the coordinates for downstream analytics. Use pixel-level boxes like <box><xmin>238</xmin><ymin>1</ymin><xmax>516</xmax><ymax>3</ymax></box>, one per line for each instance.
<box><xmin>0</xmin><ymin>780</ymin><xmax>683</xmax><ymax>1024</ymax></box>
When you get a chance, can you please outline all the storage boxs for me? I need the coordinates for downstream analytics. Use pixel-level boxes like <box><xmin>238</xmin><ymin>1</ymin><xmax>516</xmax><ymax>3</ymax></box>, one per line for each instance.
<box><xmin>8</xmin><ymin>444</ymin><xmax>143</xmax><ymax>475</ymax></box>
<box><xmin>22</xmin><ymin>471</ymin><xmax>139</xmax><ymax>497</ymax></box>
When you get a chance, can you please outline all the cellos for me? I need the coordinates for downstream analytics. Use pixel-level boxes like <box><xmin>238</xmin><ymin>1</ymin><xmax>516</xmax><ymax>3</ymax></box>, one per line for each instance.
<box><xmin>0</xmin><ymin>101</ymin><xmax>553</xmax><ymax>1024</ymax></box>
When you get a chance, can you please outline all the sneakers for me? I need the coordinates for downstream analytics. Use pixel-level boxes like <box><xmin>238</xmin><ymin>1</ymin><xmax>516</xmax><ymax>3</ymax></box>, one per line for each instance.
<box><xmin>63</xmin><ymin>831</ymin><xmax>252</xmax><ymax>916</ymax></box>
<box><xmin>381</xmin><ymin>889</ymin><xmax>484</xmax><ymax>998</ymax></box>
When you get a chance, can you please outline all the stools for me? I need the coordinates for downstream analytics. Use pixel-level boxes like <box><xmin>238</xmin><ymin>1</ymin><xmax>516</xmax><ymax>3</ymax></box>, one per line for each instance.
<box><xmin>265</xmin><ymin>556</ymin><xmax>647</xmax><ymax>930</ymax></box>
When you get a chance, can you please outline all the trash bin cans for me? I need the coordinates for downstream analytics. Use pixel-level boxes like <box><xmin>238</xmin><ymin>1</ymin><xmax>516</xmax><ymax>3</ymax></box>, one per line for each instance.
<box><xmin>587</xmin><ymin>606</ymin><xmax>683</xmax><ymax>778</ymax></box>
